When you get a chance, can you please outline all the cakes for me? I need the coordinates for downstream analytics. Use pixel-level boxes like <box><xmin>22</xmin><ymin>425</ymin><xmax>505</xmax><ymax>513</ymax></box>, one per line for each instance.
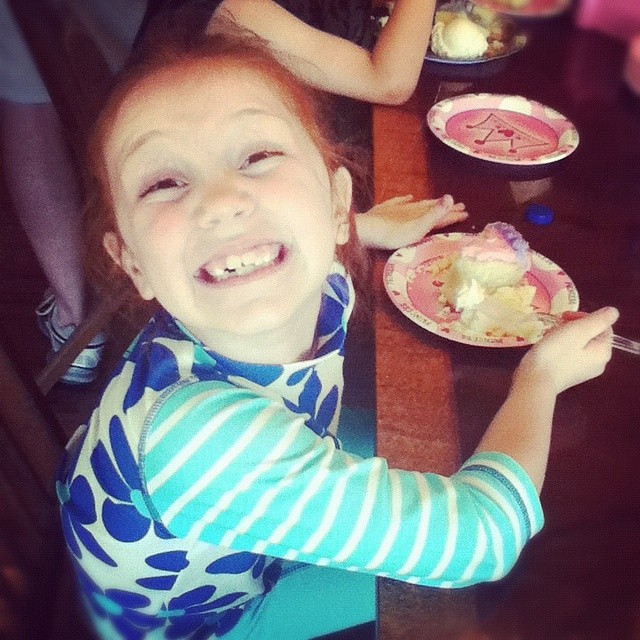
<box><xmin>432</xmin><ymin>8</ymin><xmax>487</xmax><ymax>66</ymax></box>
<box><xmin>419</xmin><ymin>220</ymin><xmax>548</xmax><ymax>346</ymax></box>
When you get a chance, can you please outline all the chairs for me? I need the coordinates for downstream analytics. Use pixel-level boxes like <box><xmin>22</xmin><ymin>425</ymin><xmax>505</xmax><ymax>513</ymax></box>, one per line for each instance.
<box><xmin>0</xmin><ymin>0</ymin><xmax>117</xmax><ymax>566</ymax></box>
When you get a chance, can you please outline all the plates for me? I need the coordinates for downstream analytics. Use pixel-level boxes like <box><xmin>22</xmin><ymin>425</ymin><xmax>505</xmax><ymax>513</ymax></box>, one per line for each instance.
<box><xmin>424</xmin><ymin>25</ymin><xmax>528</xmax><ymax>64</ymax></box>
<box><xmin>382</xmin><ymin>231</ymin><xmax>580</xmax><ymax>349</ymax></box>
<box><xmin>426</xmin><ymin>91</ymin><xmax>581</xmax><ymax>168</ymax></box>
<box><xmin>469</xmin><ymin>0</ymin><xmax>571</xmax><ymax>18</ymax></box>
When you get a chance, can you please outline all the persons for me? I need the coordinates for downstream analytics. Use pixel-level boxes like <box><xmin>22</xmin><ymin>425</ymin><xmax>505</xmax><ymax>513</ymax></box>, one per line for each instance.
<box><xmin>0</xmin><ymin>0</ymin><xmax>110</xmax><ymax>384</ymax></box>
<box><xmin>130</xmin><ymin>0</ymin><xmax>436</xmax><ymax>107</ymax></box>
<box><xmin>57</xmin><ymin>34</ymin><xmax>619</xmax><ymax>640</ymax></box>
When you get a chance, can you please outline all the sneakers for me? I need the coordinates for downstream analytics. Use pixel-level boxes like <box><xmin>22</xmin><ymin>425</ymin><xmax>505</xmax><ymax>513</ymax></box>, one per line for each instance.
<box><xmin>34</xmin><ymin>285</ymin><xmax>105</xmax><ymax>385</ymax></box>
